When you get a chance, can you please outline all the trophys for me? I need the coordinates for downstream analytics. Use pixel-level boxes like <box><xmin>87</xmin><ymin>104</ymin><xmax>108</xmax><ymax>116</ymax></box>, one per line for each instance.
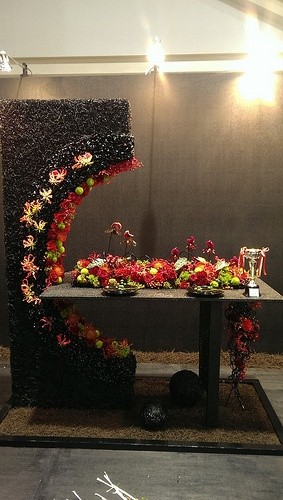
<box><xmin>241</xmin><ymin>246</ymin><xmax>270</xmax><ymax>297</ymax></box>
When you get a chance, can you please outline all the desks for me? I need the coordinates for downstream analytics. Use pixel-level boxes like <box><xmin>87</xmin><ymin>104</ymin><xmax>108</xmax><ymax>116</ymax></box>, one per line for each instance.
<box><xmin>39</xmin><ymin>276</ymin><xmax>283</xmax><ymax>428</ymax></box>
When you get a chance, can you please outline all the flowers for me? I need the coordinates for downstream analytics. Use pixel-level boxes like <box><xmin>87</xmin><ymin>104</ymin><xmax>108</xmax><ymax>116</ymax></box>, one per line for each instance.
<box><xmin>20</xmin><ymin>153</ymin><xmax>251</xmax><ymax>359</ymax></box>
<box><xmin>224</xmin><ymin>301</ymin><xmax>261</xmax><ymax>410</ymax></box>
